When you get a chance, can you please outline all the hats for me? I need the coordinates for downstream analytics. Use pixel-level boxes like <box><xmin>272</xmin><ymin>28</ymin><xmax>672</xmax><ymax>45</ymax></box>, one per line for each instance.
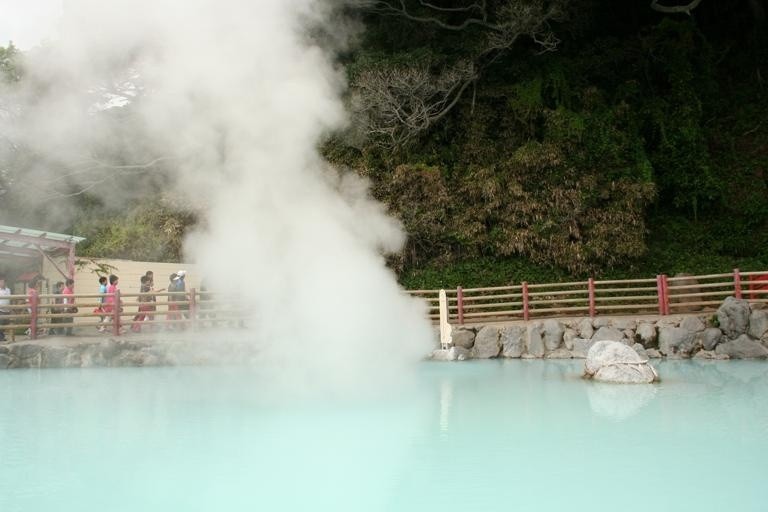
<box><xmin>177</xmin><ymin>271</ymin><xmax>186</xmax><ymax>277</ymax></box>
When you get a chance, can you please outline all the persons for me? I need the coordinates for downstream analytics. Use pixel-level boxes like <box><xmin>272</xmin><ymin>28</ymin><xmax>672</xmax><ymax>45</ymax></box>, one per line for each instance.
<box><xmin>0</xmin><ymin>279</ymin><xmax>11</xmax><ymax>340</ymax></box>
<box><xmin>62</xmin><ymin>279</ymin><xmax>75</xmax><ymax>337</ymax></box>
<box><xmin>96</xmin><ymin>271</ymin><xmax>190</xmax><ymax>336</ymax></box>
<box><xmin>24</xmin><ymin>278</ymin><xmax>40</xmax><ymax>339</ymax></box>
<box><xmin>48</xmin><ymin>282</ymin><xmax>65</xmax><ymax>336</ymax></box>
